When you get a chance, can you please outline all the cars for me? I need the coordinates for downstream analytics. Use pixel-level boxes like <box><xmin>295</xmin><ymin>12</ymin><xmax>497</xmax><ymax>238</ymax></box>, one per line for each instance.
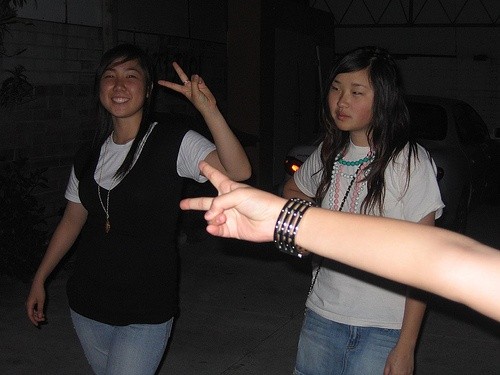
<box><xmin>280</xmin><ymin>94</ymin><xmax>494</xmax><ymax>229</ymax></box>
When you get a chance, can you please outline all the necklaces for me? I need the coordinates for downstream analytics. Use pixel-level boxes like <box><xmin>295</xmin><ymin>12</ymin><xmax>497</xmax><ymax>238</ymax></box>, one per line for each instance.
<box><xmin>95</xmin><ymin>122</ymin><xmax>152</xmax><ymax>233</ymax></box>
<box><xmin>304</xmin><ymin>146</ymin><xmax>374</xmax><ymax>214</ymax></box>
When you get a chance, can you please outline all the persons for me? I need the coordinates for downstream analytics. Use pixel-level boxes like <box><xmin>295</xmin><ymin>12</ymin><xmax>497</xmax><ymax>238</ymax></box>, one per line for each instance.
<box><xmin>179</xmin><ymin>160</ymin><xmax>500</xmax><ymax>323</ymax></box>
<box><xmin>275</xmin><ymin>45</ymin><xmax>445</xmax><ymax>375</ymax></box>
<box><xmin>26</xmin><ymin>42</ymin><xmax>252</xmax><ymax>375</ymax></box>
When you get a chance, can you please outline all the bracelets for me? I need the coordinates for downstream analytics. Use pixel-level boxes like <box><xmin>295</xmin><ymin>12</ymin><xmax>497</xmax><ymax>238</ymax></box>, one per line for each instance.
<box><xmin>273</xmin><ymin>196</ymin><xmax>315</xmax><ymax>260</ymax></box>
